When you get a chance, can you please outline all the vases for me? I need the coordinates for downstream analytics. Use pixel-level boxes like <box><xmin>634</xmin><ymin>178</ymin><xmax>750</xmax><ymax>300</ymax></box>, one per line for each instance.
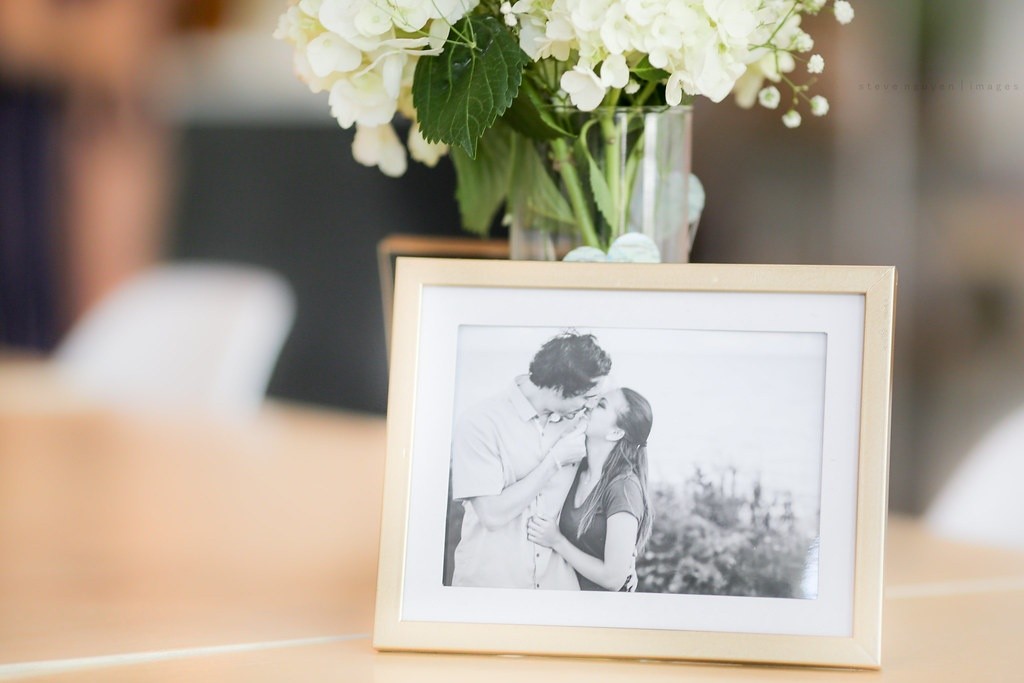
<box><xmin>506</xmin><ymin>105</ymin><xmax>693</xmax><ymax>265</ymax></box>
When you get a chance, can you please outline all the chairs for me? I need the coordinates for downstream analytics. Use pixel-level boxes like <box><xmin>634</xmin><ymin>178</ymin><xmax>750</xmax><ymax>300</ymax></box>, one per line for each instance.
<box><xmin>69</xmin><ymin>257</ymin><xmax>300</xmax><ymax>403</ymax></box>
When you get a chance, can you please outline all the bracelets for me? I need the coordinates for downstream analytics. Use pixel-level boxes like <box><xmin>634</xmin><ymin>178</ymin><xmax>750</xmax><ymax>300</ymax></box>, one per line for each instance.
<box><xmin>548</xmin><ymin>448</ymin><xmax>562</xmax><ymax>470</ymax></box>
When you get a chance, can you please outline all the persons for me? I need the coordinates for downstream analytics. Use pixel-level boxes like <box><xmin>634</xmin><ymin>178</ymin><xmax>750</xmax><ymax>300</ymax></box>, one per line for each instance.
<box><xmin>452</xmin><ymin>334</ymin><xmax>638</xmax><ymax>591</ymax></box>
<box><xmin>526</xmin><ymin>387</ymin><xmax>652</xmax><ymax>591</ymax></box>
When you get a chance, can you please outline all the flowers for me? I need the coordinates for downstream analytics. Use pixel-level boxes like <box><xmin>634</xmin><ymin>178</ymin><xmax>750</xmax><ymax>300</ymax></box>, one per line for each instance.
<box><xmin>277</xmin><ymin>0</ymin><xmax>856</xmax><ymax>172</ymax></box>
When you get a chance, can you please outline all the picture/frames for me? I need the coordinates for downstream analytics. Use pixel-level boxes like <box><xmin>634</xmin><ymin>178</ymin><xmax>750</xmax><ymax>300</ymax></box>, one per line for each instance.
<box><xmin>374</xmin><ymin>255</ymin><xmax>900</xmax><ymax>668</ymax></box>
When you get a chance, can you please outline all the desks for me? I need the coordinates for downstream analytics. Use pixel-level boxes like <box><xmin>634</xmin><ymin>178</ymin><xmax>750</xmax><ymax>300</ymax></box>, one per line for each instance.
<box><xmin>0</xmin><ymin>364</ymin><xmax>1024</xmax><ymax>683</ymax></box>
